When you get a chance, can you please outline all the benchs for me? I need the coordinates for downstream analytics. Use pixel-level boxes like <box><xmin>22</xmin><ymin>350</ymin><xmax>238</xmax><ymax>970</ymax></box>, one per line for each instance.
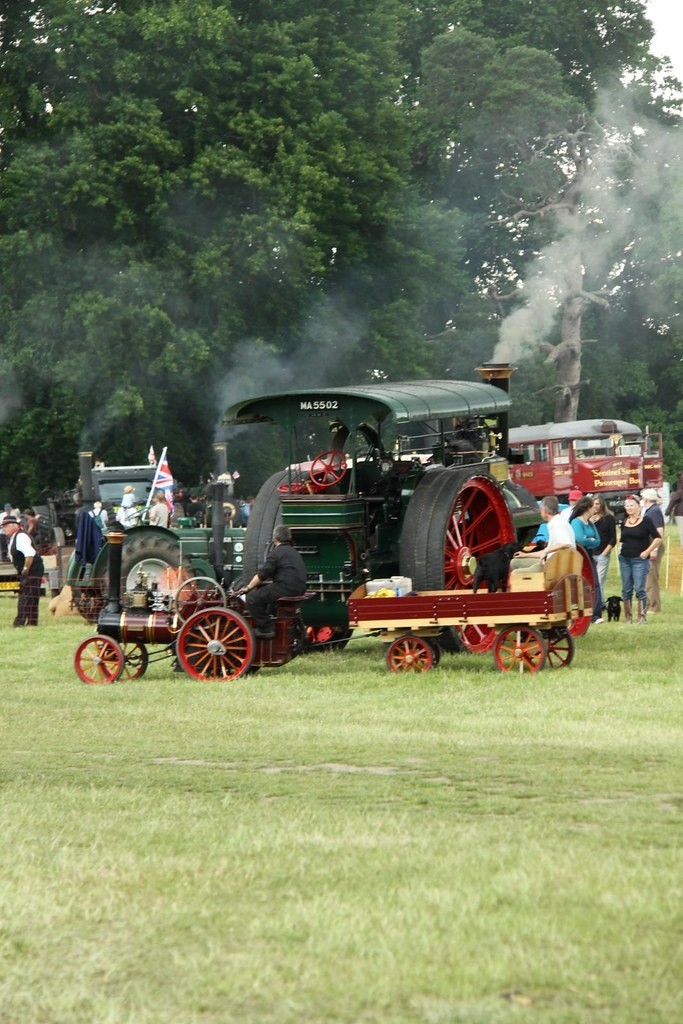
<box><xmin>510</xmin><ymin>543</ymin><xmax>585</xmax><ymax>593</ymax></box>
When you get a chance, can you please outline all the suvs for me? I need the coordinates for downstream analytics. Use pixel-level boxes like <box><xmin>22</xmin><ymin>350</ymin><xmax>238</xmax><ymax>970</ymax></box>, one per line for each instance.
<box><xmin>71</xmin><ymin>466</ymin><xmax>158</xmax><ymax>532</ymax></box>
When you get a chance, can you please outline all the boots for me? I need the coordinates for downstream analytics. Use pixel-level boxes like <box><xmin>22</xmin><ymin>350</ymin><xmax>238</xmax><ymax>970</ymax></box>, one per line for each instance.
<box><xmin>623</xmin><ymin>600</ymin><xmax>633</xmax><ymax>625</ymax></box>
<box><xmin>636</xmin><ymin>599</ymin><xmax>647</xmax><ymax>624</ymax></box>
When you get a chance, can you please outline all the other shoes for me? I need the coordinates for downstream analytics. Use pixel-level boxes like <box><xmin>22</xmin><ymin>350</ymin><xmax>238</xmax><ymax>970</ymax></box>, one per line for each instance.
<box><xmin>595</xmin><ymin>618</ymin><xmax>605</xmax><ymax>624</ymax></box>
<box><xmin>254</xmin><ymin>628</ymin><xmax>275</xmax><ymax>640</ymax></box>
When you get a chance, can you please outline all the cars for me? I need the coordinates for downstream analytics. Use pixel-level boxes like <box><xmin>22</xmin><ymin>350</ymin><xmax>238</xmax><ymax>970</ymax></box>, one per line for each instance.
<box><xmin>173</xmin><ymin>482</ymin><xmax>202</xmax><ymax>502</ymax></box>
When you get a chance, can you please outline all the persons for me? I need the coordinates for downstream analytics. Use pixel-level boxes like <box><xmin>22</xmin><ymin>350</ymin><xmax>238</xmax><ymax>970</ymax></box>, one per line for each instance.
<box><xmin>238</xmin><ymin>525</ymin><xmax>307</xmax><ymax>638</ymax></box>
<box><xmin>0</xmin><ymin>503</ymin><xmax>44</xmax><ymax>626</ymax></box>
<box><xmin>509</xmin><ymin>475</ymin><xmax>683</xmax><ymax>625</ymax></box>
<box><xmin>179</xmin><ymin>487</ymin><xmax>253</xmax><ymax>527</ymax></box>
<box><xmin>149</xmin><ymin>493</ymin><xmax>168</xmax><ymax>528</ymax></box>
<box><xmin>121</xmin><ymin>486</ymin><xmax>136</xmax><ymax>507</ymax></box>
<box><xmin>88</xmin><ymin>501</ymin><xmax>109</xmax><ymax>530</ymax></box>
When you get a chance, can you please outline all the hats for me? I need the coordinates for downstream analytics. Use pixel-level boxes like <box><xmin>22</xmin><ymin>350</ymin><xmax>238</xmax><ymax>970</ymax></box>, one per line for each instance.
<box><xmin>0</xmin><ymin>516</ymin><xmax>21</xmax><ymax>525</ymax></box>
<box><xmin>124</xmin><ymin>486</ymin><xmax>135</xmax><ymax>493</ymax></box>
<box><xmin>640</xmin><ymin>488</ymin><xmax>659</xmax><ymax>501</ymax></box>
<box><xmin>4</xmin><ymin>504</ymin><xmax>11</xmax><ymax>511</ymax></box>
<box><xmin>567</xmin><ymin>490</ymin><xmax>583</xmax><ymax>501</ymax></box>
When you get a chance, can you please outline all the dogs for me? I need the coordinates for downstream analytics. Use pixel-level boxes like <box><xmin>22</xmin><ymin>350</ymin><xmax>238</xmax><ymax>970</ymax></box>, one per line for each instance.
<box><xmin>470</xmin><ymin>540</ymin><xmax>523</xmax><ymax>594</ymax></box>
<box><xmin>604</xmin><ymin>595</ymin><xmax>626</xmax><ymax>623</ymax></box>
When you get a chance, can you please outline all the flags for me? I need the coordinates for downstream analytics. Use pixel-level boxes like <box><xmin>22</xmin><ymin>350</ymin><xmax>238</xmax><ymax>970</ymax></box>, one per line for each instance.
<box><xmin>153</xmin><ymin>454</ymin><xmax>174</xmax><ymax>517</ymax></box>
<box><xmin>148</xmin><ymin>445</ymin><xmax>155</xmax><ymax>462</ymax></box>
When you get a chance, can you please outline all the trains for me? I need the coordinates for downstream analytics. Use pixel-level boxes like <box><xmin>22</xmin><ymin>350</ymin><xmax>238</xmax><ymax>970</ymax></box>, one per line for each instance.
<box><xmin>507</xmin><ymin>419</ymin><xmax>663</xmax><ymax>523</ymax></box>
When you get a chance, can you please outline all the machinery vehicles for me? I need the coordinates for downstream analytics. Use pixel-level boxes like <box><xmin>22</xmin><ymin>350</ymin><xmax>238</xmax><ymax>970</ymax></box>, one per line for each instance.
<box><xmin>66</xmin><ymin>506</ymin><xmax>246</xmax><ymax>624</ymax></box>
<box><xmin>31</xmin><ymin>495</ymin><xmax>76</xmax><ymax>548</ymax></box>
<box><xmin>209</xmin><ymin>361</ymin><xmax>596</xmax><ymax>654</ymax></box>
